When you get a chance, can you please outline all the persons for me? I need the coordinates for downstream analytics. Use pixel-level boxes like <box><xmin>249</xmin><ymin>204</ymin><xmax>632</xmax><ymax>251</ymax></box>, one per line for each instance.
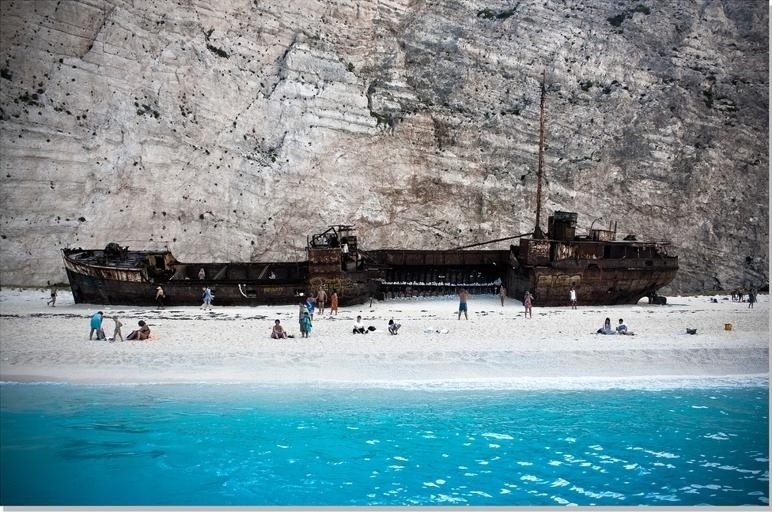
<box><xmin>457</xmin><ymin>288</ymin><xmax>471</xmax><ymax>320</ymax></box>
<box><xmin>388</xmin><ymin>319</ymin><xmax>401</xmax><ymax>335</ymax></box>
<box><xmin>523</xmin><ymin>290</ymin><xmax>534</xmax><ymax>318</ymax></box>
<box><xmin>90</xmin><ymin>311</ymin><xmax>104</xmax><ymax>340</ymax></box>
<box><xmin>598</xmin><ymin>317</ymin><xmax>616</xmax><ymax>334</ymax></box>
<box><xmin>499</xmin><ymin>284</ymin><xmax>506</xmax><ymax>307</ymax></box>
<box><xmin>747</xmin><ymin>290</ymin><xmax>754</xmax><ymax>308</ymax></box>
<box><xmin>269</xmin><ymin>272</ymin><xmax>277</xmax><ymax>279</ymax></box>
<box><xmin>112</xmin><ymin>315</ymin><xmax>124</xmax><ymax>341</ymax></box>
<box><xmin>156</xmin><ymin>286</ymin><xmax>166</xmax><ymax>310</ymax></box>
<box><xmin>198</xmin><ymin>268</ymin><xmax>206</xmax><ymax>280</ymax></box>
<box><xmin>616</xmin><ymin>319</ymin><xmax>634</xmax><ymax>335</ymax></box>
<box><xmin>299</xmin><ymin>285</ymin><xmax>341</xmax><ymax>338</ymax></box>
<box><xmin>200</xmin><ymin>287</ymin><xmax>207</xmax><ymax>309</ymax></box>
<box><xmin>569</xmin><ymin>287</ymin><xmax>577</xmax><ymax>310</ymax></box>
<box><xmin>353</xmin><ymin>315</ymin><xmax>368</xmax><ymax>335</ymax></box>
<box><xmin>127</xmin><ymin>320</ymin><xmax>151</xmax><ymax>341</ymax></box>
<box><xmin>271</xmin><ymin>319</ymin><xmax>287</xmax><ymax>339</ymax></box>
<box><xmin>731</xmin><ymin>284</ymin><xmax>757</xmax><ymax>303</ymax></box>
<box><xmin>48</xmin><ymin>283</ymin><xmax>58</xmax><ymax>307</ymax></box>
<box><xmin>202</xmin><ymin>284</ymin><xmax>214</xmax><ymax>310</ymax></box>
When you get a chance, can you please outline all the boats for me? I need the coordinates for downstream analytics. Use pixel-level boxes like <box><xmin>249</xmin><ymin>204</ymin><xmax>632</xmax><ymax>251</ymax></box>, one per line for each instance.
<box><xmin>60</xmin><ymin>69</ymin><xmax>678</xmax><ymax>309</ymax></box>
